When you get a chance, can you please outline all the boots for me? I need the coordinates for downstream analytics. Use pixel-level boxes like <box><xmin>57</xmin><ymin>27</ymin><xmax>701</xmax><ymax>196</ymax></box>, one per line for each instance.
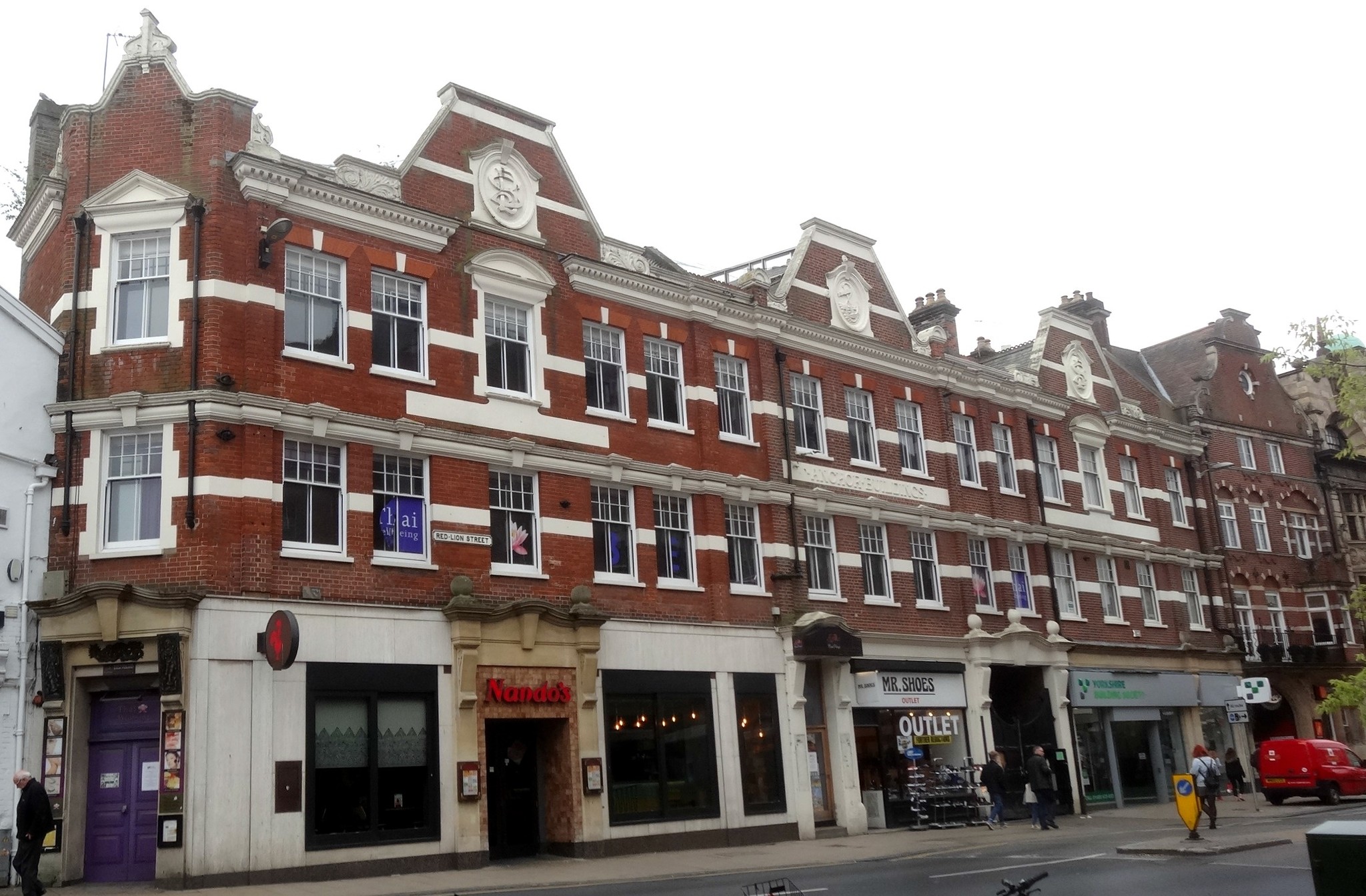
<box><xmin>1205</xmin><ymin>810</ymin><xmax>1217</xmax><ymax>824</ymax></box>
<box><xmin>1210</xmin><ymin>815</ymin><xmax>1217</xmax><ymax>829</ymax></box>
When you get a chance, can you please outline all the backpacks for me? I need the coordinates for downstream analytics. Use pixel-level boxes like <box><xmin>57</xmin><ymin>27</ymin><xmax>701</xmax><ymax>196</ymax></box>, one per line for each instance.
<box><xmin>1198</xmin><ymin>758</ymin><xmax>1220</xmax><ymax>793</ymax></box>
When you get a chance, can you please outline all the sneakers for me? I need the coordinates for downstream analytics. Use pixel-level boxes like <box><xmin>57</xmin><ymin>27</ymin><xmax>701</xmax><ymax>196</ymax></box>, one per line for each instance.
<box><xmin>986</xmin><ymin>821</ymin><xmax>994</xmax><ymax>830</ymax></box>
<box><xmin>1000</xmin><ymin>824</ymin><xmax>1009</xmax><ymax>829</ymax></box>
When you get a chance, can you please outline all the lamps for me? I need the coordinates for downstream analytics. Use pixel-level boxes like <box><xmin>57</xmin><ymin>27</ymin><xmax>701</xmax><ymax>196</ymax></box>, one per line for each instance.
<box><xmin>44</xmin><ymin>453</ymin><xmax>57</xmax><ymax>466</ymax></box>
<box><xmin>258</xmin><ymin>217</ymin><xmax>293</xmax><ymax>268</ymax></box>
<box><xmin>1196</xmin><ymin>462</ymin><xmax>1234</xmax><ymax>479</ymax></box>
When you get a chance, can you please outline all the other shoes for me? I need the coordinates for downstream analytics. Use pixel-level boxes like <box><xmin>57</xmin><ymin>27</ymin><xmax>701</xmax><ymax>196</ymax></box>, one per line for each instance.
<box><xmin>1032</xmin><ymin>825</ymin><xmax>1041</xmax><ymax>829</ymax></box>
<box><xmin>1218</xmin><ymin>797</ymin><xmax>1223</xmax><ymax>801</ymax></box>
<box><xmin>1239</xmin><ymin>795</ymin><xmax>1246</xmax><ymax>801</ymax></box>
<box><xmin>36</xmin><ymin>887</ymin><xmax>48</xmax><ymax>896</ymax></box>
<box><xmin>1236</xmin><ymin>798</ymin><xmax>1241</xmax><ymax>801</ymax></box>
<box><xmin>1046</xmin><ymin>820</ymin><xmax>1059</xmax><ymax>829</ymax></box>
<box><xmin>1042</xmin><ymin>826</ymin><xmax>1050</xmax><ymax>830</ymax></box>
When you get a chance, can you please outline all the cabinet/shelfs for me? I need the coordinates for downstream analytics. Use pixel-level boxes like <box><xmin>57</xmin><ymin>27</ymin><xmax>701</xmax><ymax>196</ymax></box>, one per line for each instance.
<box><xmin>931</xmin><ymin>756</ymin><xmax>994</xmax><ymax>828</ymax></box>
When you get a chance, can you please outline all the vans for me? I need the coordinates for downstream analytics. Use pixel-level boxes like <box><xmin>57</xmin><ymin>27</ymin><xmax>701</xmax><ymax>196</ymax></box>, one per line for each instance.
<box><xmin>1257</xmin><ymin>738</ymin><xmax>1366</xmax><ymax>806</ymax></box>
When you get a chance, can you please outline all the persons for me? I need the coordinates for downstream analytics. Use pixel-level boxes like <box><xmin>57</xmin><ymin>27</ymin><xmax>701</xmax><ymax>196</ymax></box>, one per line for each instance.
<box><xmin>1023</xmin><ymin>746</ymin><xmax>1058</xmax><ymax>830</ymax></box>
<box><xmin>1208</xmin><ymin>748</ymin><xmax>1246</xmax><ymax>801</ymax></box>
<box><xmin>165</xmin><ymin>731</ymin><xmax>180</xmax><ymax>749</ymax></box>
<box><xmin>46</xmin><ymin>737</ymin><xmax>58</xmax><ymax>755</ymax></box>
<box><xmin>980</xmin><ymin>751</ymin><xmax>1009</xmax><ymax>830</ymax></box>
<box><xmin>12</xmin><ymin>770</ymin><xmax>55</xmax><ymax>896</ymax></box>
<box><xmin>1190</xmin><ymin>745</ymin><xmax>1220</xmax><ymax>829</ymax></box>
<box><xmin>1250</xmin><ymin>744</ymin><xmax>1260</xmax><ymax>773</ymax></box>
<box><xmin>166</xmin><ymin>751</ymin><xmax>180</xmax><ymax>769</ymax></box>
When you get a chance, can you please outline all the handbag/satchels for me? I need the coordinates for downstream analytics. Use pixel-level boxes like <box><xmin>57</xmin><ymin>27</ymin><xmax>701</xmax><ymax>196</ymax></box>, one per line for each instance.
<box><xmin>1227</xmin><ymin>782</ymin><xmax>1233</xmax><ymax>794</ymax></box>
